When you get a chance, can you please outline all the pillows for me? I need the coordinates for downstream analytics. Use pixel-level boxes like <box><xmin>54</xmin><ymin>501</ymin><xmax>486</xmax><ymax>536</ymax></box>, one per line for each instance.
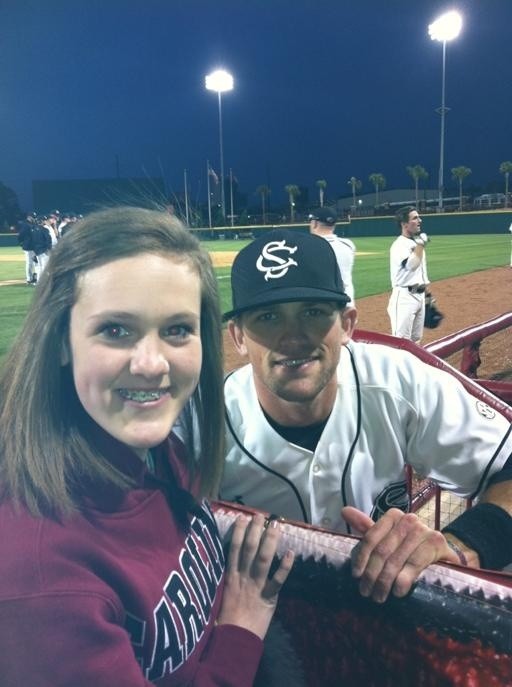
<box><xmin>425</xmin><ymin>304</ymin><xmax>443</xmax><ymax>329</ymax></box>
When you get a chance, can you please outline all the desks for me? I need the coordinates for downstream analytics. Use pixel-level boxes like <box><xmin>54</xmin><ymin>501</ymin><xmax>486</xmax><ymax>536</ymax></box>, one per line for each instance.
<box><xmin>204</xmin><ymin>66</ymin><xmax>235</xmax><ymax>220</ymax></box>
<box><xmin>426</xmin><ymin>7</ymin><xmax>464</xmax><ymax>206</ymax></box>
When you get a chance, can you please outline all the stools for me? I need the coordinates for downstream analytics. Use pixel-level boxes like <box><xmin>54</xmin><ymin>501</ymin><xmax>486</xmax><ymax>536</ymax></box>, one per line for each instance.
<box><xmin>409</xmin><ymin>286</ymin><xmax>426</xmax><ymax>293</ymax></box>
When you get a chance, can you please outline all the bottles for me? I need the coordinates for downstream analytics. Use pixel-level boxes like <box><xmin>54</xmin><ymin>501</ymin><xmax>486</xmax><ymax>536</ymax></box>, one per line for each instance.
<box><xmin>220</xmin><ymin>229</ymin><xmax>351</xmax><ymax>323</ymax></box>
<box><xmin>306</xmin><ymin>207</ymin><xmax>336</xmax><ymax>223</ymax></box>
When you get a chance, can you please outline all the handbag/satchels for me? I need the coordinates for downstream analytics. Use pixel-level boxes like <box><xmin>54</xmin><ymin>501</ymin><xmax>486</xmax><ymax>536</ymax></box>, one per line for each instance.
<box><xmin>448</xmin><ymin>540</ymin><xmax>467</xmax><ymax>565</ymax></box>
<box><xmin>451</xmin><ymin>500</ymin><xmax>512</xmax><ymax>572</ymax></box>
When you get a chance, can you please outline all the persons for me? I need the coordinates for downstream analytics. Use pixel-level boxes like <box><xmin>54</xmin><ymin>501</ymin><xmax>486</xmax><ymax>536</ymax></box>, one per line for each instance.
<box><xmin>174</xmin><ymin>228</ymin><xmax>512</xmax><ymax>604</ymax></box>
<box><xmin>1</xmin><ymin>203</ymin><xmax>297</xmax><ymax>687</ymax></box>
<box><xmin>17</xmin><ymin>210</ymin><xmax>85</xmax><ymax>285</ymax></box>
<box><xmin>164</xmin><ymin>205</ymin><xmax>177</xmax><ymax>217</ymax></box>
<box><xmin>308</xmin><ymin>206</ymin><xmax>356</xmax><ymax>309</ymax></box>
<box><xmin>387</xmin><ymin>206</ymin><xmax>435</xmax><ymax>345</ymax></box>
